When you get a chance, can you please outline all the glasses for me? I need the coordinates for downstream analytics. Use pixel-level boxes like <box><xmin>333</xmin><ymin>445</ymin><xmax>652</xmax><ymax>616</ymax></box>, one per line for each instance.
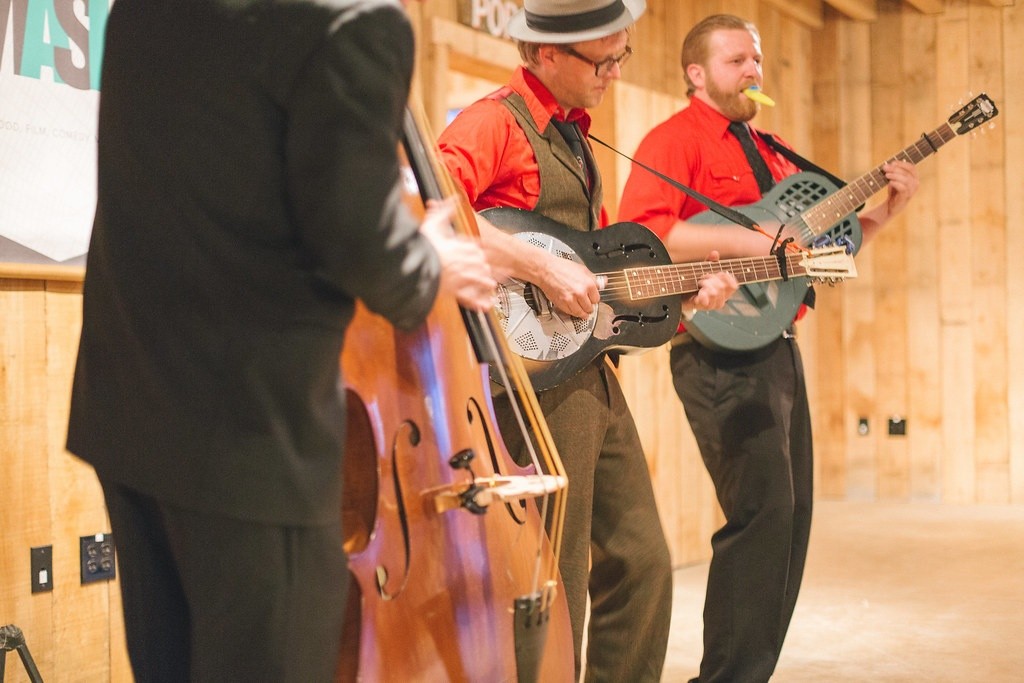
<box><xmin>561</xmin><ymin>43</ymin><xmax>633</xmax><ymax>76</ymax></box>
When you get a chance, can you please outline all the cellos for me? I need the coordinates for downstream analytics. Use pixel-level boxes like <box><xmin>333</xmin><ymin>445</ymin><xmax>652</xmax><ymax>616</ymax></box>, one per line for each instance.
<box><xmin>341</xmin><ymin>85</ymin><xmax>577</xmax><ymax>683</ymax></box>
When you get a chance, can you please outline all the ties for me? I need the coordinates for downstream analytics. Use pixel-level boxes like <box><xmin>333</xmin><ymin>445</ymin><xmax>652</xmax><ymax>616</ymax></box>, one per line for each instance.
<box><xmin>550</xmin><ymin>116</ymin><xmax>587</xmax><ymax>184</ymax></box>
<box><xmin>729</xmin><ymin>120</ymin><xmax>777</xmax><ymax>199</ymax></box>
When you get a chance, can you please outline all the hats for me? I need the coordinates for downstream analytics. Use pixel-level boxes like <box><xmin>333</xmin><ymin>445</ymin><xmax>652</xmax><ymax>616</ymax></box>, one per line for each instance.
<box><xmin>506</xmin><ymin>0</ymin><xmax>647</xmax><ymax>43</ymax></box>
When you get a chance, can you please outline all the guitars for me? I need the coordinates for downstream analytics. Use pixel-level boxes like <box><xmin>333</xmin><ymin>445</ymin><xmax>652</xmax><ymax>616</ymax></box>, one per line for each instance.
<box><xmin>475</xmin><ymin>207</ymin><xmax>857</xmax><ymax>400</ymax></box>
<box><xmin>680</xmin><ymin>93</ymin><xmax>999</xmax><ymax>358</ymax></box>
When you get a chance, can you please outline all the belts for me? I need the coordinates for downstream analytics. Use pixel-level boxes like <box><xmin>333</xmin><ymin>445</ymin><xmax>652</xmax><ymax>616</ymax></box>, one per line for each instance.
<box><xmin>670</xmin><ymin>323</ymin><xmax>798</xmax><ymax>348</ymax></box>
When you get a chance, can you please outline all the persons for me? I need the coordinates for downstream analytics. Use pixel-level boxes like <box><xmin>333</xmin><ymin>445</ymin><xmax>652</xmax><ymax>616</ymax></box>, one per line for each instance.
<box><xmin>64</xmin><ymin>0</ymin><xmax>498</xmax><ymax>683</ymax></box>
<box><xmin>436</xmin><ymin>0</ymin><xmax>740</xmax><ymax>683</ymax></box>
<box><xmin>617</xmin><ymin>14</ymin><xmax>922</xmax><ymax>683</ymax></box>
